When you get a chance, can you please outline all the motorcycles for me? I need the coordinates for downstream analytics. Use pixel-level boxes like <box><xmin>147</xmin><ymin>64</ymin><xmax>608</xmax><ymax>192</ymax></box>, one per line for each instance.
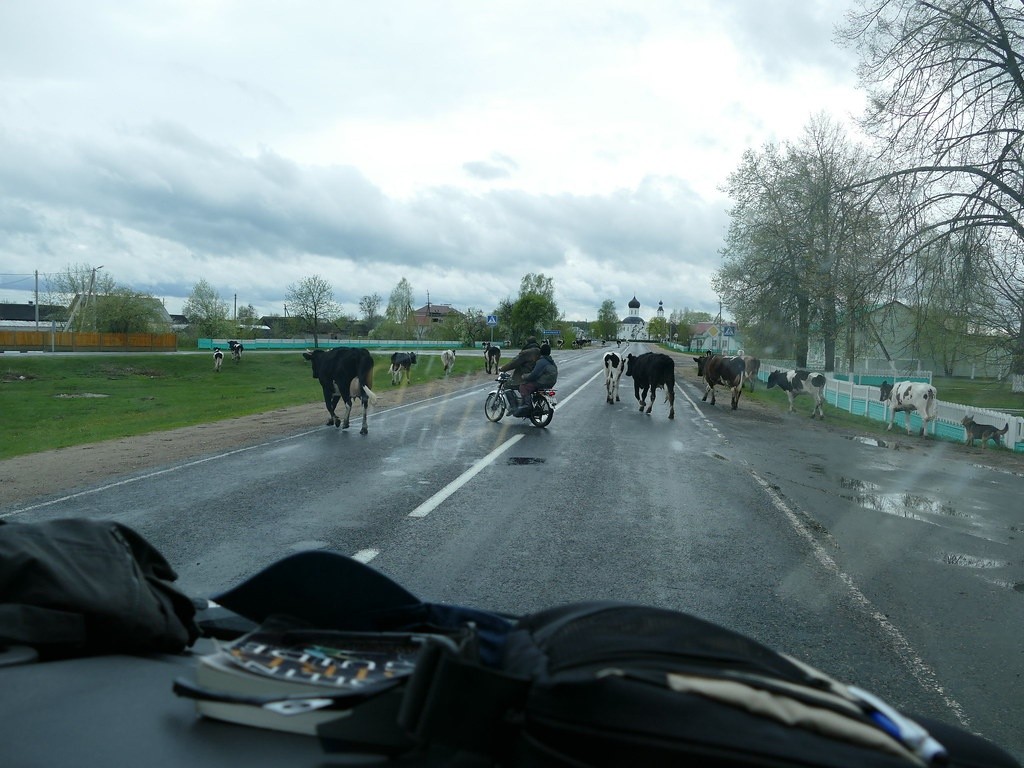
<box><xmin>484</xmin><ymin>367</ymin><xmax>558</xmax><ymax>428</ymax></box>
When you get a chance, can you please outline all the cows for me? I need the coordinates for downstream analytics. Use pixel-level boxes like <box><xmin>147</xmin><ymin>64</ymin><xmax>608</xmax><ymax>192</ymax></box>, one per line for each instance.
<box><xmin>440</xmin><ymin>349</ymin><xmax>456</xmax><ymax>376</ymax></box>
<box><xmin>482</xmin><ymin>342</ymin><xmax>501</xmax><ymax>375</ymax></box>
<box><xmin>693</xmin><ymin>353</ymin><xmax>761</xmax><ymax>410</ymax></box>
<box><xmin>227</xmin><ymin>341</ymin><xmax>243</xmax><ymax>360</ymax></box>
<box><xmin>302</xmin><ymin>347</ymin><xmax>377</xmax><ymax>434</ymax></box>
<box><xmin>603</xmin><ymin>353</ymin><xmax>626</xmax><ymax>404</ymax></box>
<box><xmin>766</xmin><ymin>369</ymin><xmax>826</xmax><ymax>419</ymax></box>
<box><xmin>879</xmin><ymin>380</ymin><xmax>937</xmax><ymax>440</ymax></box>
<box><xmin>625</xmin><ymin>352</ymin><xmax>675</xmax><ymax>419</ymax></box>
<box><xmin>212</xmin><ymin>347</ymin><xmax>224</xmax><ymax>373</ymax></box>
<box><xmin>387</xmin><ymin>352</ymin><xmax>417</xmax><ymax>386</ymax></box>
<box><xmin>540</xmin><ymin>338</ymin><xmax>592</xmax><ymax>350</ymax></box>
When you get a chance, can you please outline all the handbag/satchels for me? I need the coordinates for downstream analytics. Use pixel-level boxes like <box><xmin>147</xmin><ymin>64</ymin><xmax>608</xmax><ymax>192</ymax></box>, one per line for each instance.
<box><xmin>0</xmin><ymin>518</ymin><xmax>203</xmax><ymax>667</ymax></box>
<box><xmin>312</xmin><ymin>600</ymin><xmax>952</xmax><ymax>768</ymax></box>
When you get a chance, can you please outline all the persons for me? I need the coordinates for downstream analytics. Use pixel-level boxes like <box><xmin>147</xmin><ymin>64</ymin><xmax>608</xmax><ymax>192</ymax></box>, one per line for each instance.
<box><xmin>515</xmin><ymin>344</ymin><xmax>557</xmax><ymax>416</ymax></box>
<box><xmin>499</xmin><ymin>337</ymin><xmax>540</xmax><ymax>418</ymax></box>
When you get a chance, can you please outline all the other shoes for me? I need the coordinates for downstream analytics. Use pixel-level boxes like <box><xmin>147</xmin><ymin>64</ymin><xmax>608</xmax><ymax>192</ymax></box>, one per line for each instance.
<box><xmin>514</xmin><ymin>405</ymin><xmax>531</xmax><ymax>417</ymax></box>
<box><xmin>506</xmin><ymin>406</ymin><xmax>517</xmax><ymax>416</ymax></box>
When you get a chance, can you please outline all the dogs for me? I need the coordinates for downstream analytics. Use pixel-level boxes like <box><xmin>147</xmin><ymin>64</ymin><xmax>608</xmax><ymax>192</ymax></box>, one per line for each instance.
<box><xmin>960</xmin><ymin>415</ymin><xmax>1009</xmax><ymax>450</ymax></box>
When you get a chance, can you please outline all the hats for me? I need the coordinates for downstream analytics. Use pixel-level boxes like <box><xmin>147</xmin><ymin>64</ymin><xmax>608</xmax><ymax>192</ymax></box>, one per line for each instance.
<box><xmin>540</xmin><ymin>344</ymin><xmax>551</xmax><ymax>355</ymax></box>
<box><xmin>527</xmin><ymin>336</ymin><xmax>537</xmax><ymax>343</ymax></box>
